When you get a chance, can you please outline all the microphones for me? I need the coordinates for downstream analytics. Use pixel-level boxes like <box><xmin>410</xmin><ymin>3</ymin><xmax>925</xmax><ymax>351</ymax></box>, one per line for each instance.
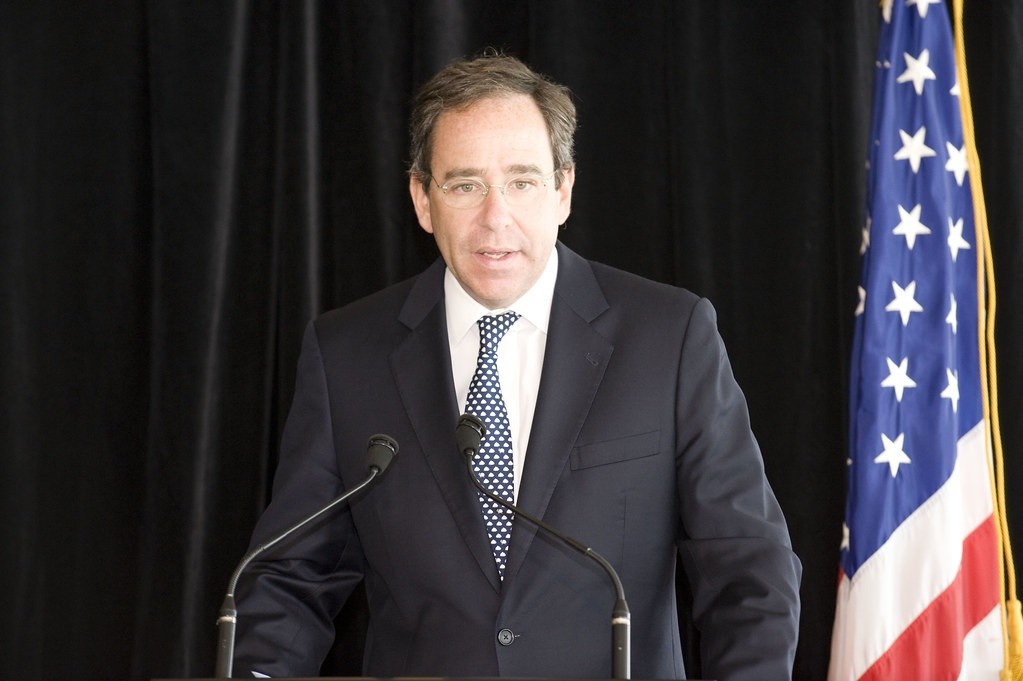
<box><xmin>215</xmin><ymin>433</ymin><xmax>400</xmax><ymax>678</ymax></box>
<box><xmin>456</xmin><ymin>414</ymin><xmax>632</xmax><ymax>680</ymax></box>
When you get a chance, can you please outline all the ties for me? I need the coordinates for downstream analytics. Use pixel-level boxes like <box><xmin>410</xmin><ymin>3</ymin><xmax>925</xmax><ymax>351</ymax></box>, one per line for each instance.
<box><xmin>465</xmin><ymin>310</ymin><xmax>523</xmax><ymax>582</ymax></box>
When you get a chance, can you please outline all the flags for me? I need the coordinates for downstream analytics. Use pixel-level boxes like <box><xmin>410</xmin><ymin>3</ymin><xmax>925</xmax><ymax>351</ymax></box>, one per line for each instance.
<box><xmin>828</xmin><ymin>0</ymin><xmax>1012</xmax><ymax>681</ymax></box>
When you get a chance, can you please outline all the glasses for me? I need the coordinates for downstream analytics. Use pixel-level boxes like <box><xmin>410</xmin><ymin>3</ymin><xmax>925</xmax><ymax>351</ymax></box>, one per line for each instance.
<box><xmin>429</xmin><ymin>169</ymin><xmax>559</xmax><ymax>210</ymax></box>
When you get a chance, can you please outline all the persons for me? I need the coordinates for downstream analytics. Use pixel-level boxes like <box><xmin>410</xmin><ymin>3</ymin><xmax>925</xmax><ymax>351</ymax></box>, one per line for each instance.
<box><xmin>234</xmin><ymin>56</ymin><xmax>803</xmax><ymax>681</ymax></box>
